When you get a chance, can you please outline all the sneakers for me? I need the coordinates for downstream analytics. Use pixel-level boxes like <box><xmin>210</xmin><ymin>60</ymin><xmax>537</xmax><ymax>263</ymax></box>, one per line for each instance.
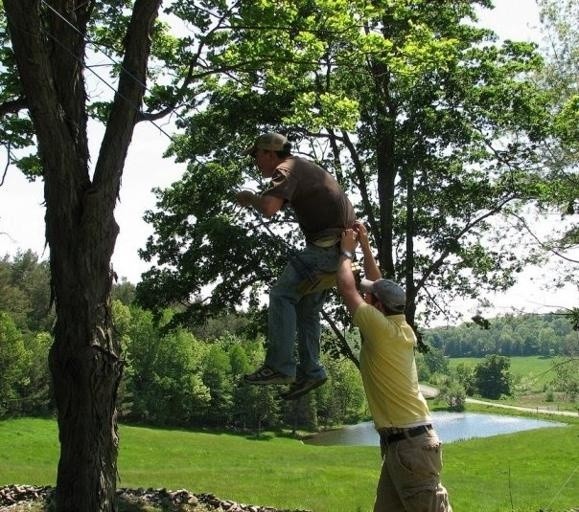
<box><xmin>283</xmin><ymin>373</ymin><xmax>326</xmax><ymax>400</ymax></box>
<box><xmin>245</xmin><ymin>365</ymin><xmax>297</xmax><ymax>384</ymax></box>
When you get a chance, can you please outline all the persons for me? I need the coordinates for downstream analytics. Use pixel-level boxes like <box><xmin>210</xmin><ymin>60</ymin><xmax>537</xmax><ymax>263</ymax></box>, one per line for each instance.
<box><xmin>336</xmin><ymin>223</ymin><xmax>452</xmax><ymax>512</ymax></box>
<box><xmin>235</xmin><ymin>132</ymin><xmax>359</xmax><ymax>399</ymax></box>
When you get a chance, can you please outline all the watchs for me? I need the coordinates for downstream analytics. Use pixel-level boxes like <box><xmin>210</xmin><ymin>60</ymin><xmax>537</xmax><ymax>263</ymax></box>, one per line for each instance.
<box><xmin>339</xmin><ymin>249</ymin><xmax>355</xmax><ymax>261</ymax></box>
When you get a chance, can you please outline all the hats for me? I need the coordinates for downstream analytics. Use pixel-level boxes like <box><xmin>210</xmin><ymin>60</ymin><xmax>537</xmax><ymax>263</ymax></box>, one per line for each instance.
<box><xmin>361</xmin><ymin>277</ymin><xmax>406</xmax><ymax>312</ymax></box>
<box><xmin>240</xmin><ymin>133</ymin><xmax>292</xmax><ymax>155</ymax></box>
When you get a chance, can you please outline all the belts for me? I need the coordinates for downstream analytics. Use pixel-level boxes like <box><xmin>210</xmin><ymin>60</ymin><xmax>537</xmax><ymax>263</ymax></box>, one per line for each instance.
<box><xmin>386</xmin><ymin>425</ymin><xmax>431</xmax><ymax>443</ymax></box>
